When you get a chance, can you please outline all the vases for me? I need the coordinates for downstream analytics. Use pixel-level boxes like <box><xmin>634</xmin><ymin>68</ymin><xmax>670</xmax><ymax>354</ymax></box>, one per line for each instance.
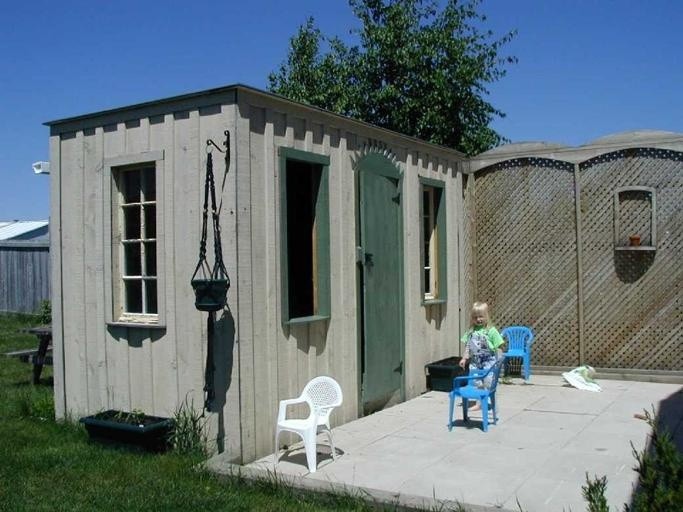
<box><xmin>425</xmin><ymin>355</ymin><xmax>469</xmax><ymax>393</ymax></box>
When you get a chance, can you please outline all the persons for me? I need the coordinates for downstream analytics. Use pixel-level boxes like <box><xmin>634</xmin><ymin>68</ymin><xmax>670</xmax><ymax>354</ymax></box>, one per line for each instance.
<box><xmin>459</xmin><ymin>302</ymin><xmax>505</xmax><ymax>412</ymax></box>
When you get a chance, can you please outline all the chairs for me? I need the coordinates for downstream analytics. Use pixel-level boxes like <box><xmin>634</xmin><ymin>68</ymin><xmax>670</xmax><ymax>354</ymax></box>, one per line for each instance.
<box><xmin>272</xmin><ymin>374</ymin><xmax>344</xmax><ymax>474</ymax></box>
<box><xmin>446</xmin><ymin>357</ymin><xmax>503</xmax><ymax>433</ymax></box>
<box><xmin>498</xmin><ymin>326</ymin><xmax>534</xmax><ymax>381</ymax></box>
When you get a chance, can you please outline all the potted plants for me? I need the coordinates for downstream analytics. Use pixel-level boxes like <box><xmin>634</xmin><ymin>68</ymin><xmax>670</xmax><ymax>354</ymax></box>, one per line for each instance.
<box><xmin>77</xmin><ymin>408</ymin><xmax>176</xmax><ymax>456</ymax></box>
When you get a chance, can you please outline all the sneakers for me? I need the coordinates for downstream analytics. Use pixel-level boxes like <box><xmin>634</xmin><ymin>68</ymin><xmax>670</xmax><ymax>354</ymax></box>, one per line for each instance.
<box><xmin>470</xmin><ymin>405</ymin><xmax>480</xmax><ymax>411</ymax></box>
